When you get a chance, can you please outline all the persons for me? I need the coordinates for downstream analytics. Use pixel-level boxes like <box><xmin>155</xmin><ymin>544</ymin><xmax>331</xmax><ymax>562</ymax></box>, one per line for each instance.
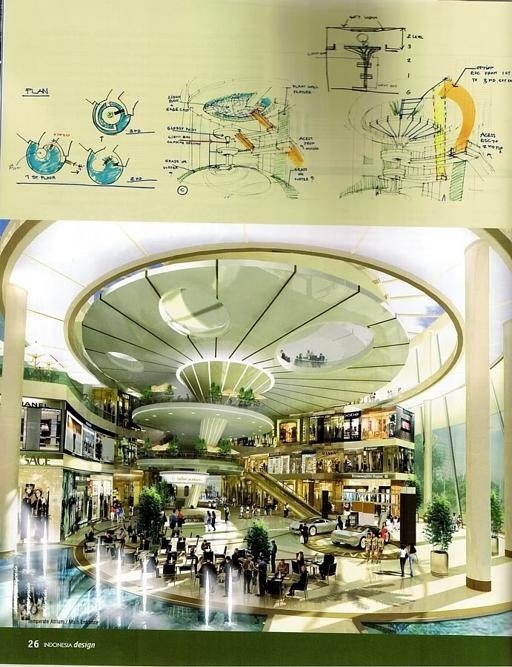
<box><xmin>13</xmin><ymin>349</ymin><xmax>425</xmax><ymax>618</ymax></box>
<box><xmin>450</xmin><ymin>512</ymin><xmax>458</xmax><ymax>531</ymax></box>
<box><xmin>456</xmin><ymin>514</ymin><xmax>462</xmax><ymax>531</ymax></box>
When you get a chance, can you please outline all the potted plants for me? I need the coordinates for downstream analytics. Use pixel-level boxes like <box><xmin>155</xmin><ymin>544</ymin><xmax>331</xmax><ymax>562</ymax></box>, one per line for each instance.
<box><xmin>424</xmin><ymin>498</ymin><xmax>460</xmax><ymax>577</ymax></box>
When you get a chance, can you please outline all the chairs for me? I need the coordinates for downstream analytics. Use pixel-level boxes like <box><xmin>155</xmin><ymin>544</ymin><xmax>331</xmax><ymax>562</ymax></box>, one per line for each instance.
<box><xmin>83</xmin><ymin>520</ymin><xmax>340</xmax><ymax>603</ymax></box>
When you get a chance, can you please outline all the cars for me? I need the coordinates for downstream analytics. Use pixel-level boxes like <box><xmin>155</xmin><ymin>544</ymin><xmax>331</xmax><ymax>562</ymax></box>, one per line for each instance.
<box><xmin>330</xmin><ymin>524</ymin><xmax>391</xmax><ymax>548</ymax></box>
<box><xmin>288</xmin><ymin>515</ymin><xmax>338</xmax><ymax>534</ymax></box>
